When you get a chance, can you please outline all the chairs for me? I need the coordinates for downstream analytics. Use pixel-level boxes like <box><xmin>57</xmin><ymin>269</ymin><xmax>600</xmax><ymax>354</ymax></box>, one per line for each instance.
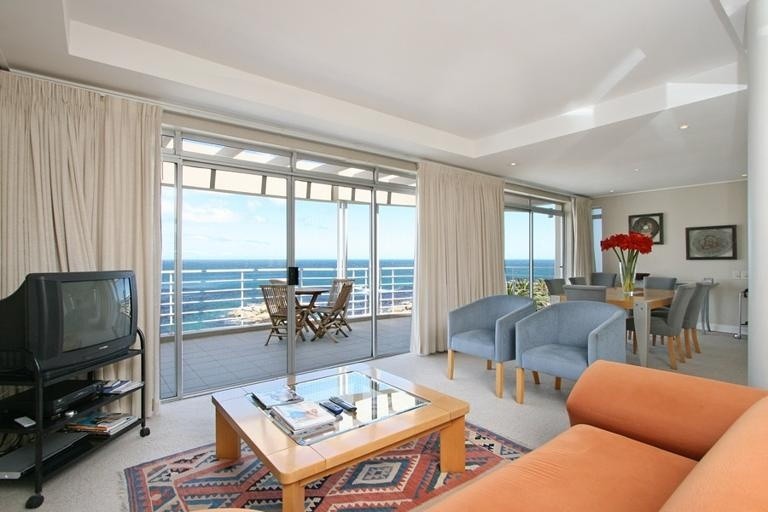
<box><xmin>589</xmin><ymin>270</ymin><xmax>616</xmax><ymax>288</ymax></box>
<box><xmin>562</xmin><ymin>288</ymin><xmax>604</xmax><ymax>301</ymax></box>
<box><xmin>545</xmin><ymin>277</ymin><xmax>568</xmax><ymax>293</ymax></box>
<box><xmin>570</xmin><ymin>275</ymin><xmax>587</xmax><ymax>287</ymax></box>
<box><xmin>515</xmin><ymin>300</ymin><xmax>626</xmax><ymax>404</ymax></box>
<box><xmin>626</xmin><ymin>282</ymin><xmax>693</xmax><ymax>369</ymax></box>
<box><xmin>655</xmin><ymin>282</ymin><xmax>706</xmax><ymax>358</ymax></box>
<box><xmin>446</xmin><ymin>293</ymin><xmax>536</xmax><ymax>398</ymax></box>
<box><xmin>645</xmin><ymin>276</ymin><xmax>674</xmax><ymax>291</ymax></box>
<box><xmin>256</xmin><ymin>275</ymin><xmax>355</xmax><ymax>343</ymax></box>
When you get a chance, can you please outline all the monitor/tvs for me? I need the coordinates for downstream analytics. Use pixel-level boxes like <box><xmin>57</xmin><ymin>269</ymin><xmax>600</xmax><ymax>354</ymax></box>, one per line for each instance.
<box><xmin>0</xmin><ymin>269</ymin><xmax>138</xmax><ymax>374</ymax></box>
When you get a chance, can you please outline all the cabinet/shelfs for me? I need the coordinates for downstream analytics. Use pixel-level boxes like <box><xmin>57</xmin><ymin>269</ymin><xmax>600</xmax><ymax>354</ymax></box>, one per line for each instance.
<box><xmin>0</xmin><ymin>329</ymin><xmax>149</xmax><ymax>507</ymax></box>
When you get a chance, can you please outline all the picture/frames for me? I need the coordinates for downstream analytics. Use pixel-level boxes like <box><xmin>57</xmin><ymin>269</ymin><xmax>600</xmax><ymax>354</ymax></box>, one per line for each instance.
<box><xmin>685</xmin><ymin>224</ymin><xmax>737</xmax><ymax>260</ymax></box>
<box><xmin>629</xmin><ymin>212</ymin><xmax>664</xmax><ymax>244</ymax></box>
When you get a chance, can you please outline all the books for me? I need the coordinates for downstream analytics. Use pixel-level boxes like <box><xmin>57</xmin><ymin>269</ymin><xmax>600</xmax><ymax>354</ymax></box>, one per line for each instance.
<box><xmin>270</xmin><ymin>400</ymin><xmax>336</xmax><ymax>439</ymax></box>
<box><xmin>63</xmin><ymin>411</ymin><xmax>138</xmax><ymax>436</ymax></box>
<box><xmin>252</xmin><ymin>386</ymin><xmax>304</xmax><ymax>409</ymax></box>
<box><xmin>99</xmin><ymin>379</ymin><xmax>143</xmax><ymax>395</ymax></box>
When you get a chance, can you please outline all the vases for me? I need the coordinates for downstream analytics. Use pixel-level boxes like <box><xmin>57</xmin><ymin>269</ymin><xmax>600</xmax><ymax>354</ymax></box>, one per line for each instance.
<box><xmin>619</xmin><ymin>261</ymin><xmax>637</xmax><ymax>294</ymax></box>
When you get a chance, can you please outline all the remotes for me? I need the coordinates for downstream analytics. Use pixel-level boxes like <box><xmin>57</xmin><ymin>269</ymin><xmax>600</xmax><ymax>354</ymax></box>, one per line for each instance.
<box><xmin>319</xmin><ymin>400</ymin><xmax>342</xmax><ymax>414</ymax></box>
<box><xmin>330</xmin><ymin>396</ymin><xmax>357</xmax><ymax>412</ymax></box>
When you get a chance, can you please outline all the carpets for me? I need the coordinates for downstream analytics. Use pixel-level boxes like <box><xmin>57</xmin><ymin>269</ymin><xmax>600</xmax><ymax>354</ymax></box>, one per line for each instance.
<box><xmin>123</xmin><ymin>411</ymin><xmax>534</xmax><ymax>511</ymax></box>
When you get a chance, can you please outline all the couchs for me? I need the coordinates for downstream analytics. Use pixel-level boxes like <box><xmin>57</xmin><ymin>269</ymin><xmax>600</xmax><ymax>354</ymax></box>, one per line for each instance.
<box><xmin>194</xmin><ymin>359</ymin><xmax>767</xmax><ymax>512</ymax></box>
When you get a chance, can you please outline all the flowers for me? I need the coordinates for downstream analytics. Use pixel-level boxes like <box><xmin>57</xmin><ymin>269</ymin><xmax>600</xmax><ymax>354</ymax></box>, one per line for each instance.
<box><xmin>600</xmin><ymin>233</ymin><xmax>654</xmax><ymax>289</ymax></box>
